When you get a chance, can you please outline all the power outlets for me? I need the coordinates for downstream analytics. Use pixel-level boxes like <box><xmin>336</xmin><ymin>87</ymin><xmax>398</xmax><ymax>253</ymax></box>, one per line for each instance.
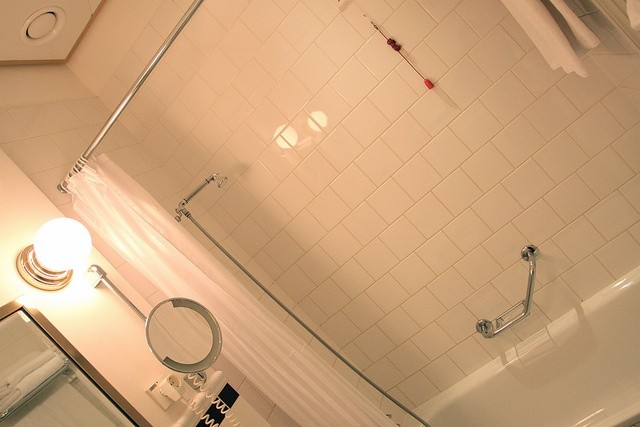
<box><xmin>145</xmin><ymin>375</ymin><xmax>186</xmax><ymax>409</ymax></box>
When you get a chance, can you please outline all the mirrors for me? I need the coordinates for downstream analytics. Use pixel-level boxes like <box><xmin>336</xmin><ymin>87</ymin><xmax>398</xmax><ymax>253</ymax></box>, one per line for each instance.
<box><xmin>146</xmin><ymin>296</ymin><xmax>223</xmax><ymax>373</ymax></box>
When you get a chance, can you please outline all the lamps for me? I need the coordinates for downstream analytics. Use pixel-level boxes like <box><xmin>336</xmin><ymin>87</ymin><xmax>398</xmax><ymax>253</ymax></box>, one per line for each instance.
<box><xmin>16</xmin><ymin>216</ymin><xmax>92</xmax><ymax>291</ymax></box>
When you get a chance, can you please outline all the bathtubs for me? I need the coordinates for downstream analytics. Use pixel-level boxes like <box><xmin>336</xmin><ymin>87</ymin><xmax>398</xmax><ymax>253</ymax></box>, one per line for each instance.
<box><xmin>401</xmin><ymin>266</ymin><xmax>639</xmax><ymax>426</ymax></box>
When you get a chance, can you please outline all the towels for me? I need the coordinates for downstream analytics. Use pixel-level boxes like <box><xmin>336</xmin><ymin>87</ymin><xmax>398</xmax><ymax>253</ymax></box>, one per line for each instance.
<box><xmin>501</xmin><ymin>1</ymin><xmax>640</xmax><ymax>78</ymax></box>
<box><xmin>1</xmin><ymin>349</ymin><xmax>57</xmax><ymax>399</ymax></box>
<box><xmin>1</xmin><ymin>355</ymin><xmax>65</xmax><ymax>416</ymax></box>
